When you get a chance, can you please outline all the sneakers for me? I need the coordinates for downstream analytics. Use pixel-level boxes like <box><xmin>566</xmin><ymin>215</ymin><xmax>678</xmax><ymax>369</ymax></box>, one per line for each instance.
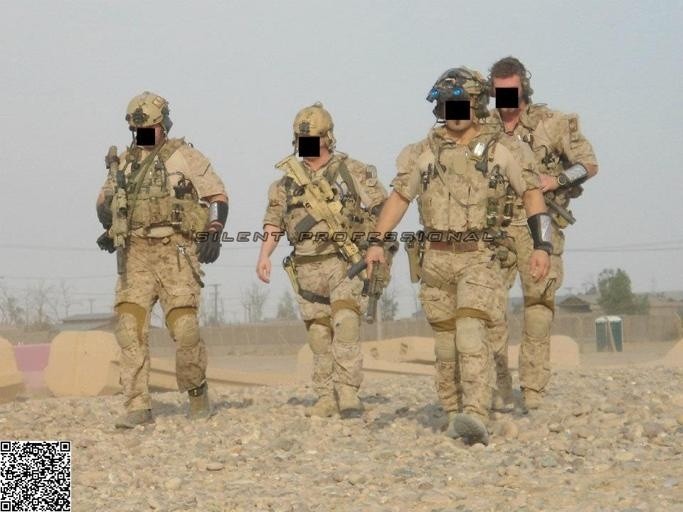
<box><xmin>188</xmin><ymin>384</ymin><xmax>213</xmax><ymax>425</ymax></box>
<box><xmin>304</xmin><ymin>386</ymin><xmax>364</xmax><ymax>418</ymax></box>
<box><xmin>115</xmin><ymin>408</ymin><xmax>156</xmax><ymax>428</ymax></box>
<box><xmin>493</xmin><ymin>386</ymin><xmax>540</xmax><ymax>414</ymax></box>
<box><xmin>445</xmin><ymin>409</ymin><xmax>488</xmax><ymax>445</ymax></box>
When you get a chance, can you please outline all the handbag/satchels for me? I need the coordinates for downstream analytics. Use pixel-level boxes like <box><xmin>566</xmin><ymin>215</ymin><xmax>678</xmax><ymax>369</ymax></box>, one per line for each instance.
<box><xmin>405</xmin><ymin>237</ymin><xmax>420</xmax><ymax>283</ymax></box>
<box><xmin>96</xmin><ymin>233</ymin><xmax>115</xmax><ymax>253</ymax></box>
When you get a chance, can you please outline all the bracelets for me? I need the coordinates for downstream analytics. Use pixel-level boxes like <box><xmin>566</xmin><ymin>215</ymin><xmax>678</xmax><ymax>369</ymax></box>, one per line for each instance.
<box><xmin>368</xmin><ymin>235</ymin><xmax>384</xmax><ymax>246</ymax></box>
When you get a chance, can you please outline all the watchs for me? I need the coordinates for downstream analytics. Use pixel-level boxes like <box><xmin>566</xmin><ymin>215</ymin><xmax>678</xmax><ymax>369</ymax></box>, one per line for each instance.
<box><xmin>557</xmin><ymin>174</ymin><xmax>569</xmax><ymax>188</ymax></box>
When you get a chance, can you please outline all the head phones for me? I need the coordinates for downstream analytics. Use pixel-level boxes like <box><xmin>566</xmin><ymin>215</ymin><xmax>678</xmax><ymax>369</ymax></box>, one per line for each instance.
<box><xmin>487</xmin><ymin>58</ymin><xmax>530</xmax><ymax>97</ymax></box>
<box><xmin>432</xmin><ymin>67</ymin><xmax>490</xmax><ymax>119</ymax></box>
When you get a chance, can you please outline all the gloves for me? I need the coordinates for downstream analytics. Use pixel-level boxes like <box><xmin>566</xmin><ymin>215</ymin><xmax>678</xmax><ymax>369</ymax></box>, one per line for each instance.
<box><xmin>97</xmin><ymin>204</ymin><xmax>113</xmax><ymax>229</ymax></box>
<box><xmin>195</xmin><ymin>230</ymin><xmax>220</xmax><ymax>264</ymax></box>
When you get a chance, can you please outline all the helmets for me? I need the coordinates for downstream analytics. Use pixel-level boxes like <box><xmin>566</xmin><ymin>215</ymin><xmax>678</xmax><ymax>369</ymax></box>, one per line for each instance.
<box><xmin>426</xmin><ymin>68</ymin><xmax>486</xmax><ymax>120</ymax></box>
<box><xmin>293</xmin><ymin>102</ymin><xmax>334</xmax><ymax>137</ymax></box>
<box><xmin>127</xmin><ymin>91</ymin><xmax>168</xmax><ymax>130</ymax></box>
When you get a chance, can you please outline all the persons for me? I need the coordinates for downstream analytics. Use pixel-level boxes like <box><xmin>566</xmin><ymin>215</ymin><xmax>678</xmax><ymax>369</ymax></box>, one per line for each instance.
<box><xmin>365</xmin><ymin>67</ymin><xmax>555</xmax><ymax>446</ymax></box>
<box><xmin>480</xmin><ymin>55</ymin><xmax>599</xmax><ymax>412</ymax></box>
<box><xmin>255</xmin><ymin>101</ymin><xmax>400</xmax><ymax>417</ymax></box>
<box><xmin>97</xmin><ymin>91</ymin><xmax>229</xmax><ymax>428</ymax></box>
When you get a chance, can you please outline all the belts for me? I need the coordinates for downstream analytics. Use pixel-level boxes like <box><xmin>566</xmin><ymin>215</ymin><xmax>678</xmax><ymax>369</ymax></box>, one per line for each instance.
<box><xmin>429</xmin><ymin>241</ymin><xmax>477</xmax><ymax>252</ymax></box>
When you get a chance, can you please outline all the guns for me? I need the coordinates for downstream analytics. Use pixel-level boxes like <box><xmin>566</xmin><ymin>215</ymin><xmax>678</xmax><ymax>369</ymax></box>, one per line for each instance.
<box><xmin>275</xmin><ymin>154</ymin><xmax>369</xmax><ymax>297</ymax></box>
<box><xmin>543</xmin><ymin>186</ymin><xmax>576</xmax><ymax>225</ymax></box>
<box><xmin>347</xmin><ymin>230</ymin><xmax>399</xmax><ymax>323</ymax></box>
<box><xmin>97</xmin><ymin>145</ymin><xmax>129</xmax><ymax>276</ymax></box>
